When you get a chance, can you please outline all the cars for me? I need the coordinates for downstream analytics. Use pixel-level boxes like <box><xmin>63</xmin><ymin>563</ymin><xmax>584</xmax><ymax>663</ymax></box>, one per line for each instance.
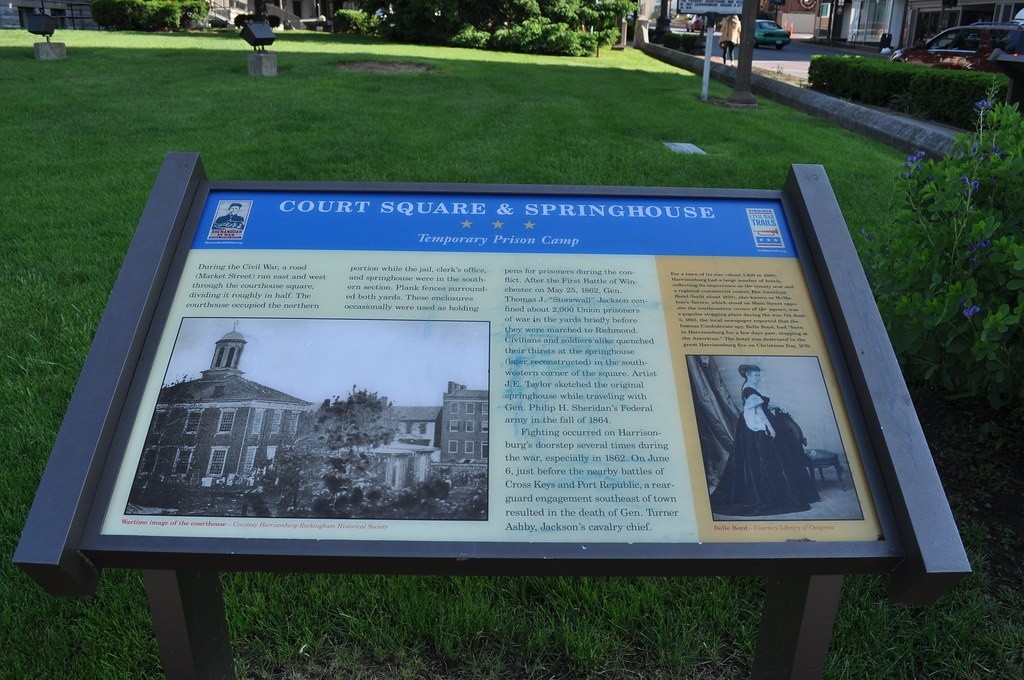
<box><xmin>684</xmin><ymin>13</ymin><xmax>709</xmax><ymax>33</ymax></box>
<box><xmin>752</xmin><ymin>20</ymin><xmax>791</xmax><ymax>50</ymax></box>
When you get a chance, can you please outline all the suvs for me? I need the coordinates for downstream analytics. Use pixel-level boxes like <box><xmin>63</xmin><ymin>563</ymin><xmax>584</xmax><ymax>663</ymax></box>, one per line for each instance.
<box><xmin>889</xmin><ymin>19</ymin><xmax>1024</xmax><ymax>71</ymax></box>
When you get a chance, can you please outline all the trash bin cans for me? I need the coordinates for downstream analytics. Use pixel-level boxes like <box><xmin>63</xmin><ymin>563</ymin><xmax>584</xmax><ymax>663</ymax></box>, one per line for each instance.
<box><xmin>877</xmin><ymin>32</ymin><xmax>892</xmax><ymax>55</ymax></box>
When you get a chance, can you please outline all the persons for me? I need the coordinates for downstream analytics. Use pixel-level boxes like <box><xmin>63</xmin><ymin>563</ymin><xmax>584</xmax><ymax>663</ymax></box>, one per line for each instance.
<box><xmin>738</xmin><ymin>364</ymin><xmax>781</xmax><ymax>506</ymax></box>
<box><xmin>212</xmin><ymin>203</ymin><xmax>244</xmax><ymax>229</ymax></box>
<box><xmin>719</xmin><ymin>15</ymin><xmax>741</xmax><ymax>66</ymax></box>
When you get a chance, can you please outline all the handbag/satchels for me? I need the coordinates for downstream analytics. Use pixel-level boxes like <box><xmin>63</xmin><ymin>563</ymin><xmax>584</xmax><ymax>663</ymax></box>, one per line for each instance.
<box><xmin>881</xmin><ymin>47</ymin><xmax>892</xmax><ymax>55</ymax></box>
